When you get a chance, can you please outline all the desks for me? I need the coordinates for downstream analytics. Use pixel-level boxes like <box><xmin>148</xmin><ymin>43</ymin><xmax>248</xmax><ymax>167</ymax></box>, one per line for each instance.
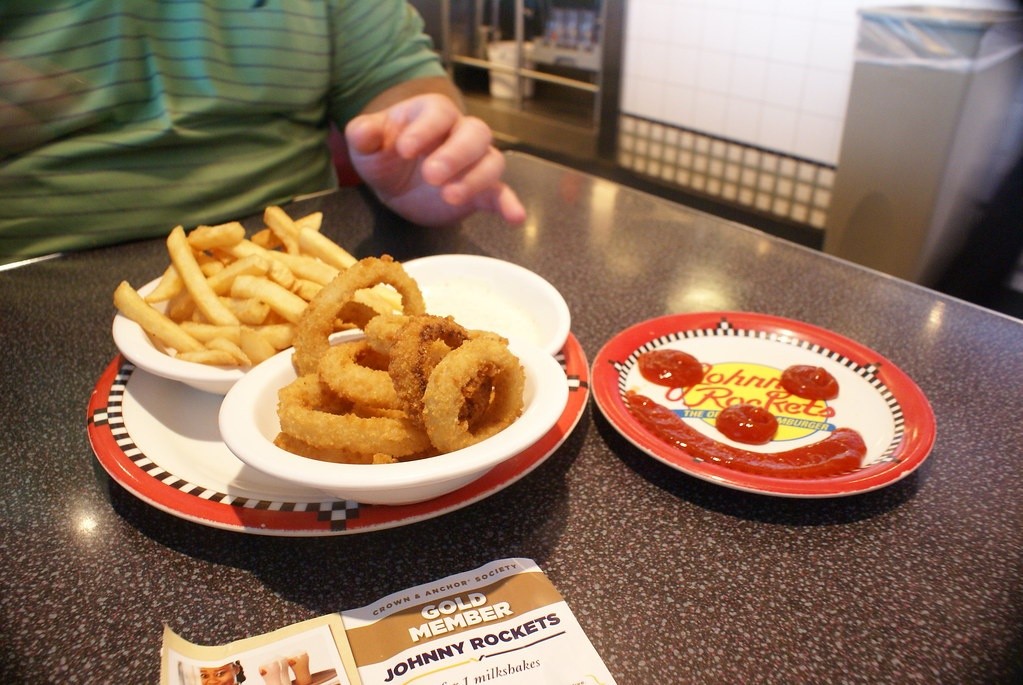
<box><xmin>1</xmin><ymin>149</ymin><xmax>1023</xmax><ymax>685</ymax></box>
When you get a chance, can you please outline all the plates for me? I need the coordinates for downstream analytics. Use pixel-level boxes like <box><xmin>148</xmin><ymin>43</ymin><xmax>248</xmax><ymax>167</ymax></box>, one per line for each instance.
<box><xmin>218</xmin><ymin>330</ymin><xmax>569</xmax><ymax>505</ymax></box>
<box><xmin>86</xmin><ymin>329</ymin><xmax>591</xmax><ymax>537</ymax></box>
<box><xmin>112</xmin><ymin>275</ymin><xmax>256</xmax><ymax>396</ymax></box>
<box><xmin>388</xmin><ymin>253</ymin><xmax>572</xmax><ymax>358</ymax></box>
<box><xmin>590</xmin><ymin>311</ymin><xmax>936</xmax><ymax>498</ymax></box>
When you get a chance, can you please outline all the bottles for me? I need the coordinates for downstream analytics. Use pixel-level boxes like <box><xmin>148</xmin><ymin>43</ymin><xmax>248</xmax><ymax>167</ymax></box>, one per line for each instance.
<box><xmin>543</xmin><ymin>8</ymin><xmax>596</xmax><ymax>50</ymax></box>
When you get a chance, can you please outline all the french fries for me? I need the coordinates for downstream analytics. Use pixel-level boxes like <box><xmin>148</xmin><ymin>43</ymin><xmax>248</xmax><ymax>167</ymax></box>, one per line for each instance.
<box><xmin>112</xmin><ymin>205</ymin><xmax>409</xmax><ymax>370</ymax></box>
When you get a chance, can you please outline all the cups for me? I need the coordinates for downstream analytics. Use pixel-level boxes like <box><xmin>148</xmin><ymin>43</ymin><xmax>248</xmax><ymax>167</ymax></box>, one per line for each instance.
<box><xmin>258</xmin><ymin>652</ymin><xmax>313</xmax><ymax>685</ymax></box>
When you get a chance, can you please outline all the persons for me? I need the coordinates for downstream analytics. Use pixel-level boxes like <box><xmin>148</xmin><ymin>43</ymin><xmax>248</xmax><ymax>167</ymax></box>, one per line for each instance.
<box><xmin>0</xmin><ymin>0</ymin><xmax>526</xmax><ymax>266</ymax></box>
